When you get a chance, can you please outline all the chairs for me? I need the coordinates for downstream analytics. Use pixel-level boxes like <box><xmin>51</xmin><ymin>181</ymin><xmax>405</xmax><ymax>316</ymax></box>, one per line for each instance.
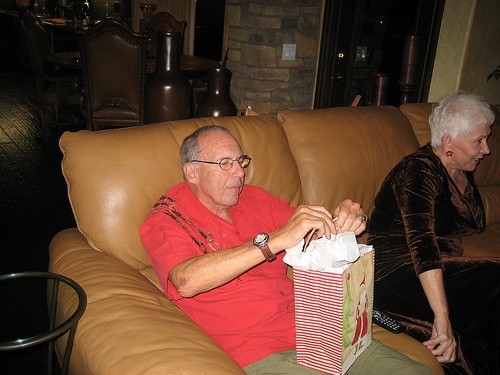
<box><xmin>19</xmin><ymin>7</ymin><xmax>86</xmax><ymax>138</ymax></box>
<box><xmin>140</xmin><ymin>12</ymin><xmax>187</xmax><ymax>54</ymax></box>
<box><xmin>77</xmin><ymin>19</ymin><xmax>147</xmax><ymax>130</ymax></box>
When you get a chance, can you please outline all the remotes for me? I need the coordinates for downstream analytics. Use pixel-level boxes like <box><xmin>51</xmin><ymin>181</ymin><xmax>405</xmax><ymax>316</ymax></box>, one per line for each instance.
<box><xmin>372</xmin><ymin>310</ymin><xmax>405</xmax><ymax>334</ymax></box>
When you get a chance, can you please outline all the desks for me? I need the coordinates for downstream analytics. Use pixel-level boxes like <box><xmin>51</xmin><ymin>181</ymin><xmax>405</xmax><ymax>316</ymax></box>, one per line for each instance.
<box><xmin>47</xmin><ymin>51</ymin><xmax>217</xmax><ymax>79</ymax></box>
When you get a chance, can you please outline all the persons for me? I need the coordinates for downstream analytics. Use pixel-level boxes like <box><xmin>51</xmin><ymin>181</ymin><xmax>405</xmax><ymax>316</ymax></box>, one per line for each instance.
<box><xmin>367</xmin><ymin>93</ymin><xmax>500</xmax><ymax>375</ymax></box>
<box><xmin>139</xmin><ymin>125</ymin><xmax>433</xmax><ymax>375</ymax></box>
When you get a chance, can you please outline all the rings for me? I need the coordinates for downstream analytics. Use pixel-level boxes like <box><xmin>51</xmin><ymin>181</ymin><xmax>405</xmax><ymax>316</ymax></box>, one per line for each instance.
<box><xmin>356</xmin><ymin>213</ymin><xmax>366</xmax><ymax>221</ymax></box>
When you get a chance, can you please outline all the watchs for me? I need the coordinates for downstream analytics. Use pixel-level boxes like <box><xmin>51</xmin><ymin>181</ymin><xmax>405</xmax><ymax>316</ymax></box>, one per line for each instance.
<box><xmin>254</xmin><ymin>232</ymin><xmax>276</xmax><ymax>262</ymax></box>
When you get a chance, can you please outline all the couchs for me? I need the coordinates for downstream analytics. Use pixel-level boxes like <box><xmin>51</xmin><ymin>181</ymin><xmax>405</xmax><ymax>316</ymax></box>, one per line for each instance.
<box><xmin>46</xmin><ymin>102</ymin><xmax>500</xmax><ymax>375</ymax></box>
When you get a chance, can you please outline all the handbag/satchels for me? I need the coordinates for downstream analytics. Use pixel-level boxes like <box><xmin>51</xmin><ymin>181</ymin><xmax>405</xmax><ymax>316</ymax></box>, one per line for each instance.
<box><xmin>292</xmin><ymin>217</ymin><xmax>375</xmax><ymax>375</ymax></box>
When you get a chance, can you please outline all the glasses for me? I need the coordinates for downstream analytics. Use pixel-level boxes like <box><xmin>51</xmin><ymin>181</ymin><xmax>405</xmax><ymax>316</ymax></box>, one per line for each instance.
<box><xmin>190</xmin><ymin>155</ymin><xmax>252</xmax><ymax>171</ymax></box>
